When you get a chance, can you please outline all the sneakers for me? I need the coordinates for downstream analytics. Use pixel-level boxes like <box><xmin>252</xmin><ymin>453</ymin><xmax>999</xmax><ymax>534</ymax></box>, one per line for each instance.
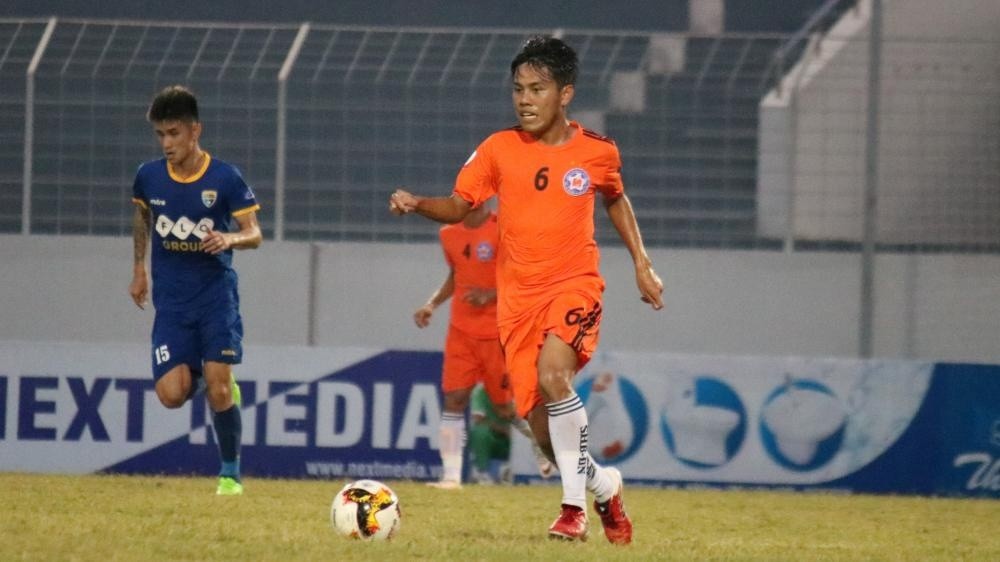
<box><xmin>594</xmin><ymin>467</ymin><xmax>634</xmax><ymax>547</ymax></box>
<box><xmin>548</xmin><ymin>505</ymin><xmax>592</xmax><ymax>540</ymax></box>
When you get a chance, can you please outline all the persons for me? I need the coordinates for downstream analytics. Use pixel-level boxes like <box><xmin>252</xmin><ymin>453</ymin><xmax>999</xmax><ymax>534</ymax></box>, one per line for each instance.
<box><xmin>411</xmin><ymin>202</ymin><xmax>555</xmax><ymax>491</ymax></box>
<box><xmin>130</xmin><ymin>87</ymin><xmax>264</xmax><ymax>495</ymax></box>
<box><xmin>388</xmin><ymin>34</ymin><xmax>664</xmax><ymax>545</ymax></box>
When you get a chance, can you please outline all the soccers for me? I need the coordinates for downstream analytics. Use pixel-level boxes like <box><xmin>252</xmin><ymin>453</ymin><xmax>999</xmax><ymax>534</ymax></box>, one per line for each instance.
<box><xmin>330</xmin><ymin>479</ymin><xmax>403</xmax><ymax>544</ymax></box>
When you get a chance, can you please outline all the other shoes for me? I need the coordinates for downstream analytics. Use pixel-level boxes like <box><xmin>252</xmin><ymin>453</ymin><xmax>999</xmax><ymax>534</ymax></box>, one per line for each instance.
<box><xmin>231</xmin><ymin>375</ymin><xmax>241</xmax><ymax>407</ymax></box>
<box><xmin>531</xmin><ymin>443</ymin><xmax>555</xmax><ymax>478</ymax></box>
<box><xmin>215</xmin><ymin>474</ymin><xmax>244</xmax><ymax>496</ymax></box>
<box><xmin>426</xmin><ymin>479</ymin><xmax>462</xmax><ymax>492</ymax></box>
